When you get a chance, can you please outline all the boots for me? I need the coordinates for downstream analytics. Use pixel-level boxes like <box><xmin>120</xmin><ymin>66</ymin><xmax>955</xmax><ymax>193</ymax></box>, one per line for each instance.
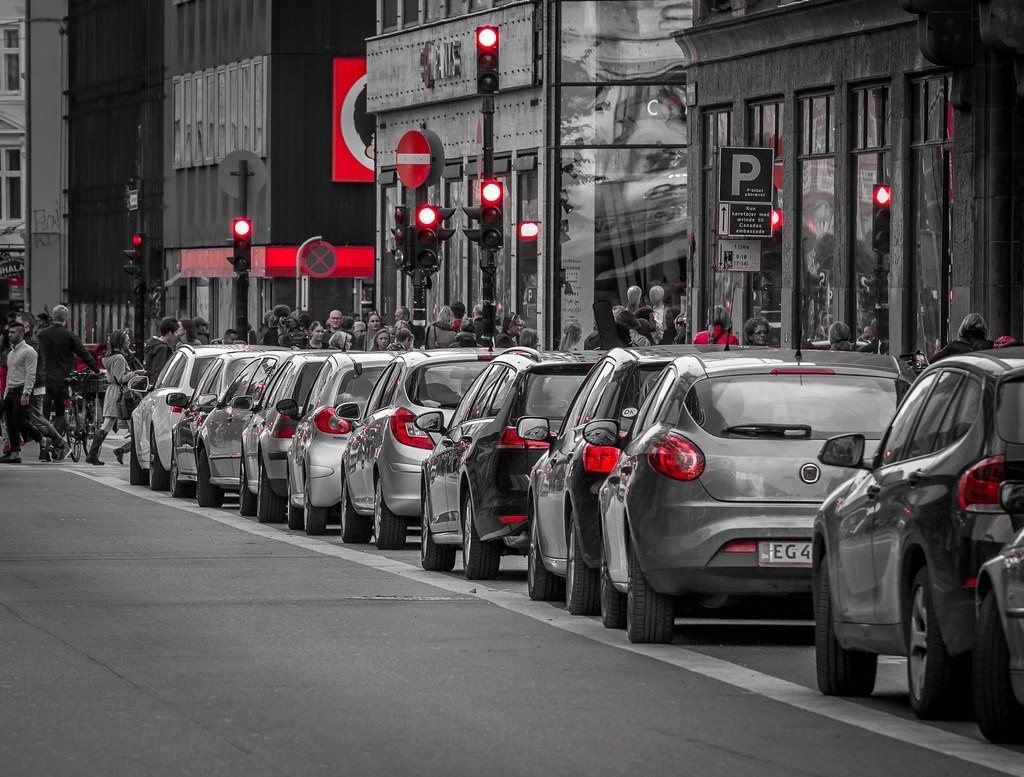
<box><xmin>85</xmin><ymin>428</ymin><xmax>108</xmax><ymax>465</ymax></box>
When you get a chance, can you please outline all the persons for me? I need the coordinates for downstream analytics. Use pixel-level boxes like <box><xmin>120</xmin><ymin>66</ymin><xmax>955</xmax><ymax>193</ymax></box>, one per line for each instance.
<box><xmin>0</xmin><ymin>321</ymin><xmax>53</xmax><ymax>464</ymax></box>
<box><xmin>86</xmin><ymin>329</ymin><xmax>147</xmax><ymax>466</ymax></box>
<box><xmin>929</xmin><ymin>313</ymin><xmax>994</xmax><ymax>365</ymax></box>
<box><xmin>1</xmin><ymin>299</ymin><xmax>886</xmax><ymax>466</ymax></box>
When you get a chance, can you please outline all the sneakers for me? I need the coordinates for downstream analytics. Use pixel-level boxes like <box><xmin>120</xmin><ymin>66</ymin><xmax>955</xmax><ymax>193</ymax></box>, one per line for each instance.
<box><xmin>52</xmin><ymin>442</ymin><xmax>73</xmax><ymax>463</ymax></box>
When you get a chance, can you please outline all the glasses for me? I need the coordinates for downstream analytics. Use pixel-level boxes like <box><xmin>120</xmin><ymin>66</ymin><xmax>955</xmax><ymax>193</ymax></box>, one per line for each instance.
<box><xmin>368</xmin><ymin>311</ymin><xmax>378</xmax><ymax>315</ymax></box>
<box><xmin>676</xmin><ymin>321</ymin><xmax>684</xmax><ymax>326</ymax></box>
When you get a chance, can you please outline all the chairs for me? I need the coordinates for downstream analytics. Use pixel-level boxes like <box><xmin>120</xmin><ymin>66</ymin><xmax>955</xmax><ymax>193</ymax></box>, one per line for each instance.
<box><xmin>712</xmin><ymin>384</ymin><xmax>744</xmax><ymax>424</ymax></box>
<box><xmin>346</xmin><ymin>379</ymin><xmax>372</xmax><ymax>398</ymax></box>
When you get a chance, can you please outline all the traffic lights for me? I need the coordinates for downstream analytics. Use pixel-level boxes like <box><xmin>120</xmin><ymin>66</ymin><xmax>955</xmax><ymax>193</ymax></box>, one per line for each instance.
<box><xmin>772</xmin><ymin>210</ymin><xmax>782</xmax><ymax>236</ymax></box>
<box><xmin>122</xmin><ymin>233</ymin><xmax>141</xmax><ymax>275</ymax></box>
<box><xmin>873</xmin><ymin>184</ymin><xmax>889</xmax><ymax>255</ymax></box>
<box><xmin>476</xmin><ymin>26</ymin><xmax>499</xmax><ymax>94</ymax></box>
<box><xmin>520</xmin><ymin>222</ymin><xmax>537</xmax><ymax>273</ymax></box>
<box><xmin>233</xmin><ymin>219</ymin><xmax>251</xmax><ymax>273</ymax></box>
<box><xmin>462</xmin><ymin>182</ymin><xmax>503</xmax><ymax>251</ymax></box>
<box><xmin>395</xmin><ymin>206</ymin><xmax>407</xmax><ymax>268</ymax></box>
<box><xmin>415</xmin><ymin>202</ymin><xmax>456</xmax><ymax>269</ymax></box>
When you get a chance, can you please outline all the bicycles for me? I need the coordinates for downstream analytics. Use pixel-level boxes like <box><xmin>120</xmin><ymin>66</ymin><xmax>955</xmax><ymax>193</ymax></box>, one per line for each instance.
<box><xmin>63</xmin><ymin>370</ymin><xmax>102</xmax><ymax>463</ymax></box>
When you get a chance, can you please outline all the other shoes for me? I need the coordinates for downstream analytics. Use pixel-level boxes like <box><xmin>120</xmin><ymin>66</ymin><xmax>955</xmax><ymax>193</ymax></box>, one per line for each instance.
<box><xmin>114</xmin><ymin>448</ymin><xmax>124</xmax><ymax>464</ymax></box>
<box><xmin>0</xmin><ymin>455</ymin><xmax>21</xmax><ymax>463</ymax></box>
<box><xmin>39</xmin><ymin>438</ymin><xmax>52</xmax><ymax>461</ymax></box>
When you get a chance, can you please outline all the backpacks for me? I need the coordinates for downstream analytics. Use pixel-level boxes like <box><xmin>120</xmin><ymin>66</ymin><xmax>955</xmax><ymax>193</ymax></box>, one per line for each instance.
<box><xmin>277</xmin><ymin>317</ymin><xmax>311</xmax><ymax>347</ymax></box>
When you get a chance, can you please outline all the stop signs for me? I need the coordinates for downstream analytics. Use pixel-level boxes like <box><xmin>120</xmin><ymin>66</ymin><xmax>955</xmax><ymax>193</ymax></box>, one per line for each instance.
<box><xmin>395</xmin><ymin>131</ymin><xmax>432</xmax><ymax>188</ymax></box>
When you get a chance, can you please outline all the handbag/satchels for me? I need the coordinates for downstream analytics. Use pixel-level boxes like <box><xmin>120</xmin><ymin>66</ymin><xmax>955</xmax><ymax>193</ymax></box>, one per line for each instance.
<box><xmin>117</xmin><ymin>390</ymin><xmax>142</xmax><ymax>419</ymax></box>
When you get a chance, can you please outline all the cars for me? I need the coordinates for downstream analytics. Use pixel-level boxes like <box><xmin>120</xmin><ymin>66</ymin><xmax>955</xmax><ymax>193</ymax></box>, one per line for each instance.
<box><xmin>582</xmin><ymin>349</ymin><xmax>920</xmax><ymax>643</ymax></box>
<box><xmin>968</xmin><ymin>481</ymin><xmax>1024</xmax><ymax>748</ymax></box>
<box><xmin>164</xmin><ymin>351</ymin><xmax>336</xmax><ymax>523</ymax></box>
<box><xmin>335</xmin><ymin>348</ymin><xmax>511</xmax><ymax>550</ymax></box>
<box><xmin>810</xmin><ymin>346</ymin><xmax>1023</xmax><ymax>723</ymax></box>
<box><xmin>516</xmin><ymin>348</ymin><xmax>784</xmax><ymax>615</ymax></box>
<box><xmin>286</xmin><ymin>350</ymin><xmax>407</xmax><ymax>536</ymax></box>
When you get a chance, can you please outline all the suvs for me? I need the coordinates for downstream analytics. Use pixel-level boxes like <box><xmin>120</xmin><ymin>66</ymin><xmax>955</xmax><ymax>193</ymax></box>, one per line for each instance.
<box><xmin>419</xmin><ymin>347</ymin><xmax>602</xmax><ymax>580</ymax></box>
<box><xmin>125</xmin><ymin>344</ymin><xmax>302</xmax><ymax>490</ymax></box>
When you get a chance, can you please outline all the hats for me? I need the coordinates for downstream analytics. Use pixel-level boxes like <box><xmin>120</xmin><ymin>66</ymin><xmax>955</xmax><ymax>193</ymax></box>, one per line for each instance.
<box><xmin>263</xmin><ymin>310</ymin><xmax>273</xmax><ymax>324</ymax></box>
<box><xmin>192</xmin><ymin>317</ymin><xmax>210</xmax><ymax>326</ymax></box>
<box><xmin>355</xmin><ymin>322</ymin><xmax>365</xmax><ymax>328</ymax></box>
<box><xmin>461</xmin><ymin>320</ymin><xmax>475</xmax><ymax>332</ymax></box>
<box><xmin>450</xmin><ymin>319</ymin><xmax>461</xmax><ymax>329</ymax></box>
<box><xmin>451</xmin><ymin>300</ymin><xmax>465</xmax><ymax>311</ymax></box>
<box><xmin>274</xmin><ymin>305</ymin><xmax>291</xmax><ymax>318</ymax></box>
